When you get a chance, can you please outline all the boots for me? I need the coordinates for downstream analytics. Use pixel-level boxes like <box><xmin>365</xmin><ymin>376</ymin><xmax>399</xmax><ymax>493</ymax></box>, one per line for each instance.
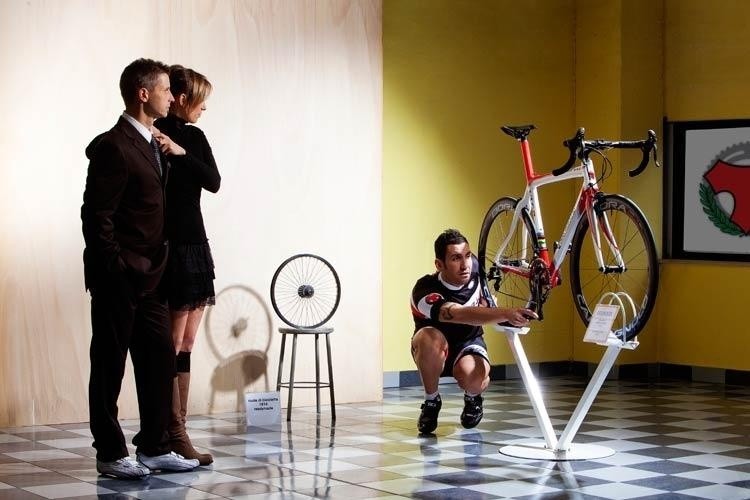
<box><xmin>172</xmin><ymin>371</ymin><xmax>214</xmax><ymax>466</ymax></box>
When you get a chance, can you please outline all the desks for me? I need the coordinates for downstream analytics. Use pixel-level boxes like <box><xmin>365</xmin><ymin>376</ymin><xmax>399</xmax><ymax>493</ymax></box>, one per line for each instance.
<box><xmin>276</xmin><ymin>325</ymin><xmax>337</xmax><ymax>424</ymax></box>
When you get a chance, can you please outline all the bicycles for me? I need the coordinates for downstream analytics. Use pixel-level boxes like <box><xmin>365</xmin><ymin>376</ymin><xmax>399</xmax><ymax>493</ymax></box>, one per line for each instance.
<box><xmin>478</xmin><ymin>125</ymin><xmax>660</xmax><ymax>342</ymax></box>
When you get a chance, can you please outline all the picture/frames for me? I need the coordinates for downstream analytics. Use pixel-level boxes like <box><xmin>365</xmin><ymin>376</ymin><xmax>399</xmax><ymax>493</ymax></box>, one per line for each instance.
<box><xmin>661</xmin><ymin>115</ymin><xmax>748</xmax><ymax>262</ymax></box>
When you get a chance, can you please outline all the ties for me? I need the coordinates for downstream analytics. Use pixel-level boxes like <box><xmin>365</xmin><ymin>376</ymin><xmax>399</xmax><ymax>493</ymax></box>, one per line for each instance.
<box><xmin>151</xmin><ymin>133</ymin><xmax>164</xmax><ymax>182</ymax></box>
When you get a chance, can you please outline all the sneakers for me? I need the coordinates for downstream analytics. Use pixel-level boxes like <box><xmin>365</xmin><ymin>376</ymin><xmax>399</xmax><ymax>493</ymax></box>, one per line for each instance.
<box><xmin>136</xmin><ymin>450</ymin><xmax>200</xmax><ymax>471</ymax></box>
<box><xmin>416</xmin><ymin>393</ymin><xmax>442</xmax><ymax>433</ymax></box>
<box><xmin>460</xmin><ymin>393</ymin><xmax>483</xmax><ymax>429</ymax></box>
<box><xmin>96</xmin><ymin>455</ymin><xmax>150</xmax><ymax>479</ymax></box>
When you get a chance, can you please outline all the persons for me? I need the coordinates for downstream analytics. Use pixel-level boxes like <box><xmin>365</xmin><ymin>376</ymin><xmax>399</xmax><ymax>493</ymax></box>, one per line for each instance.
<box><xmin>410</xmin><ymin>229</ymin><xmax>539</xmax><ymax>433</ymax></box>
<box><xmin>81</xmin><ymin>57</ymin><xmax>199</xmax><ymax>480</ymax></box>
